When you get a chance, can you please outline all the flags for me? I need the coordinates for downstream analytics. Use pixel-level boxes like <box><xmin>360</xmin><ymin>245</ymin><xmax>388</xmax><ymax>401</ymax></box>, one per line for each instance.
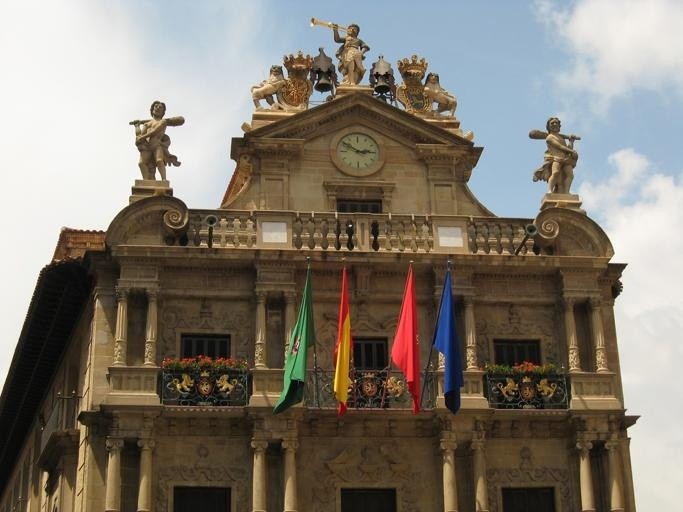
<box><xmin>270</xmin><ymin>254</ymin><xmax>316</xmax><ymax>417</ymax></box>
<box><xmin>389</xmin><ymin>258</ymin><xmax>423</xmax><ymax>416</ymax></box>
<box><xmin>332</xmin><ymin>255</ymin><xmax>355</xmax><ymax>419</ymax></box>
<box><xmin>431</xmin><ymin>260</ymin><xmax>464</xmax><ymax>415</ymax></box>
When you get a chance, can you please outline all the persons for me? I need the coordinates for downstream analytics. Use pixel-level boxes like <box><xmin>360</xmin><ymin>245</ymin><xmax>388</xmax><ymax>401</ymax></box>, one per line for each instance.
<box><xmin>132</xmin><ymin>101</ymin><xmax>169</xmax><ymax>183</ymax></box>
<box><xmin>530</xmin><ymin>116</ymin><xmax>580</xmax><ymax>198</ymax></box>
<box><xmin>328</xmin><ymin>21</ymin><xmax>370</xmax><ymax>87</ymax></box>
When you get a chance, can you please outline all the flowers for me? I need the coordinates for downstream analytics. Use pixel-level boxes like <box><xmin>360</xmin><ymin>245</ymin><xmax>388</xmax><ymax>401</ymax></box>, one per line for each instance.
<box><xmin>486</xmin><ymin>360</ymin><xmax>564</xmax><ymax>378</ymax></box>
<box><xmin>160</xmin><ymin>355</ymin><xmax>246</xmax><ymax>375</ymax></box>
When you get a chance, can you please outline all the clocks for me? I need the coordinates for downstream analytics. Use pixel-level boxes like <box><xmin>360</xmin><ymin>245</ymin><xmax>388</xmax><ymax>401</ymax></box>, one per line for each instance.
<box><xmin>330</xmin><ymin>126</ymin><xmax>387</xmax><ymax>178</ymax></box>
